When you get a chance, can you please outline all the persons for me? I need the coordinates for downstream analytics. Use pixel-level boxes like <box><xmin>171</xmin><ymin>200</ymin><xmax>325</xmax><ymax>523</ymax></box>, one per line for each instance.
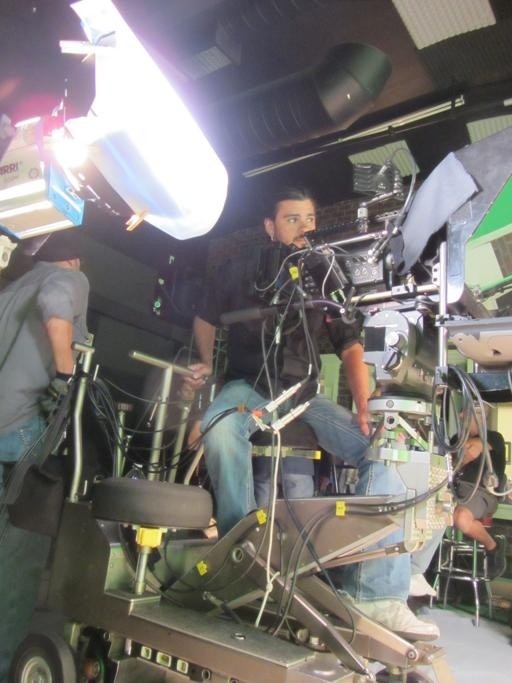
<box><xmin>179</xmin><ymin>178</ymin><xmax>442</xmax><ymax>642</ymax></box>
<box><xmin>450</xmin><ymin>395</ymin><xmax>509</xmax><ymax>582</ymax></box>
<box><xmin>0</xmin><ymin>235</ymin><xmax>95</xmax><ymax>486</ymax></box>
<box><xmin>138</xmin><ymin>334</ymin><xmax>206</xmax><ymax>486</ymax></box>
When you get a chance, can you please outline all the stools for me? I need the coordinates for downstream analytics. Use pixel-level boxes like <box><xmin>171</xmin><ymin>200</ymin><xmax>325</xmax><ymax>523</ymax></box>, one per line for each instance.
<box><xmin>429</xmin><ymin>512</ymin><xmax>492</xmax><ymax>632</ymax></box>
<box><xmin>249</xmin><ymin>417</ymin><xmax>321</xmax><ymax>459</ymax></box>
<box><xmin>94</xmin><ymin>476</ymin><xmax>213</xmax><ymax>596</ymax></box>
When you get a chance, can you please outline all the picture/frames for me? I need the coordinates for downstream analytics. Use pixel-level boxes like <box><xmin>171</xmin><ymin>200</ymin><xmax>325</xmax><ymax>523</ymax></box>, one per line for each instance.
<box><xmin>504</xmin><ymin>441</ymin><xmax>511</xmax><ymax>465</ymax></box>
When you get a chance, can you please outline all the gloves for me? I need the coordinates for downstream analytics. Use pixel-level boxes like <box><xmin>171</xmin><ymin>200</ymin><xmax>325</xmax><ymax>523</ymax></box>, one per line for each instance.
<box><xmin>40</xmin><ymin>372</ymin><xmax>76</xmax><ymax>422</ymax></box>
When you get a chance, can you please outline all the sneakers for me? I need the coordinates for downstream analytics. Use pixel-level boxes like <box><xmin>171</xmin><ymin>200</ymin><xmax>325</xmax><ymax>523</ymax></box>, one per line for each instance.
<box><xmin>353</xmin><ymin>597</ymin><xmax>440</xmax><ymax>642</ymax></box>
<box><xmin>409</xmin><ymin>575</ymin><xmax>439</xmax><ymax>597</ymax></box>
<box><xmin>486</xmin><ymin>535</ymin><xmax>507</xmax><ymax>580</ymax></box>
